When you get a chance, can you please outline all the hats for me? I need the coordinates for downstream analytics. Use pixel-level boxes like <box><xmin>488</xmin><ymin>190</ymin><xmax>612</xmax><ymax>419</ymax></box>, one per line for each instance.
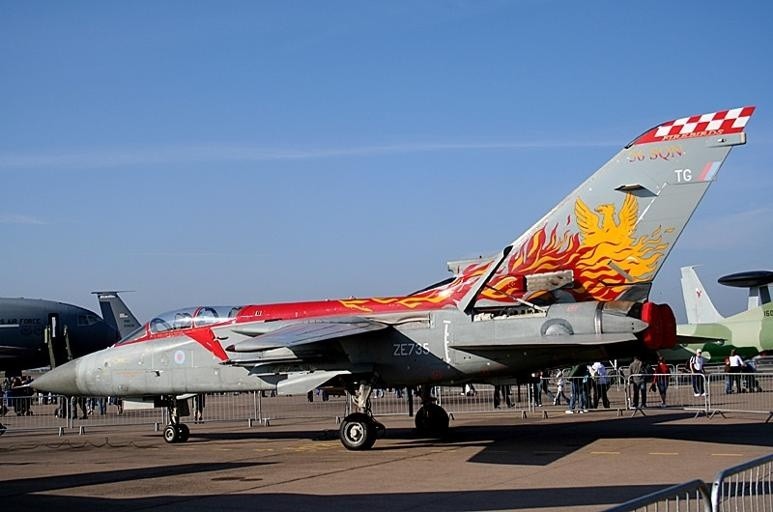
<box><xmin>697</xmin><ymin>348</ymin><xmax>703</xmax><ymax>354</ymax></box>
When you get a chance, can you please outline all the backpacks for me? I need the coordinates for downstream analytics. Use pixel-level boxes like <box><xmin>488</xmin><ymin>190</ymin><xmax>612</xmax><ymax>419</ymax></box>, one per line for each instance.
<box><xmin>569</xmin><ymin>364</ymin><xmax>584</xmax><ymax>379</ymax></box>
<box><xmin>686</xmin><ymin>356</ymin><xmax>696</xmax><ymax>369</ymax></box>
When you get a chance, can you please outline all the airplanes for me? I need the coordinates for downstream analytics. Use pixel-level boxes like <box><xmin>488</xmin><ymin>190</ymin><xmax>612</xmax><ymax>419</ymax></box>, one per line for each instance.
<box><xmin>567</xmin><ymin>263</ymin><xmax>772</xmax><ymax>379</ymax></box>
<box><xmin>9</xmin><ymin>105</ymin><xmax>753</xmax><ymax>452</ymax></box>
<box><xmin>0</xmin><ymin>289</ymin><xmax>144</xmax><ymax>373</ymax></box>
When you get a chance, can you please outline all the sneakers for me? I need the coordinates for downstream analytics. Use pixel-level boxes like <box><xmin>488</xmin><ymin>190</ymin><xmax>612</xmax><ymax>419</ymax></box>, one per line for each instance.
<box><xmin>657</xmin><ymin>403</ymin><xmax>666</xmax><ymax>407</ymax></box>
<box><xmin>577</xmin><ymin>409</ymin><xmax>588</xmax><ymax>414</ymax></box>
<box><xmin>630</xmin><ymin>406</ymin><xmax>637</xmax><ymax>409</ymax></box>
<box><xmin>565</xmin><ymin>409</ymin><xmax>574</xmax><ymax>413</ymax></box>
<box><xmin>694</xmin><ymin>392</ymin><xmax>707</xmax><ymax>397</ymax></box>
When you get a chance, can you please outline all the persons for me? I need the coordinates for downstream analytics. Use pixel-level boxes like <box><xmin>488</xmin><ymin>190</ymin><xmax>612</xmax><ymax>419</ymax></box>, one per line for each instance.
<box><xmin>628</xmin><ymin>357</ymin><xmax>669</xmax><ymax>408</ymax></box>
<box><xmin>0</xmin><ymin>375</ymin><xmax>34</xmax><ymax>429</ymax></box>
<box><xmin>49</xmin><ymin>392</ymin><xmax>57</xmax><ymax>404</ymax></box>
<box><xmin>261</xmin><ymin>390</ymin><xmax>275</xmax><ymax>397</ymax></box>
<box><xmin>460</xmin><ymin>384</ymin><xmax>477</xmax><ymax>396</ymax></box>
<box><xmin>527</xmin><ymin>362</ymin><xmax>610</xmax><ymax>414</ymax></box>
<box><xmin>690</xmin><ymin>349</ymin><xmax>707</xmax><ymax>397</ymax></box>
<box><xmin>192</xmin><ymin>394</ymin><xmax>205</xmax><ymax>424</ymax></box>
<box><xmin>372</xmin><ymin>389</ymin><xmax>384</xmax><ymax>399</ymax></box>
<box><xmin>725</xmin><ymin>350</ymin><xmax>762</xmax><ymax>393</ymax></box>
<box><xmin>54</xmin><ymin>395</ymin><xmax>123</xmax><ymax>419</ymax></box>
<box><xmin>493</xmin><ymin>385</ymin><xmax>515</xmax><ymax>409</ymax></box>
<box><xmin>44</xmin><ymin>394</ymin><xmax>48</xmax><ymax>405</ymax></box>
